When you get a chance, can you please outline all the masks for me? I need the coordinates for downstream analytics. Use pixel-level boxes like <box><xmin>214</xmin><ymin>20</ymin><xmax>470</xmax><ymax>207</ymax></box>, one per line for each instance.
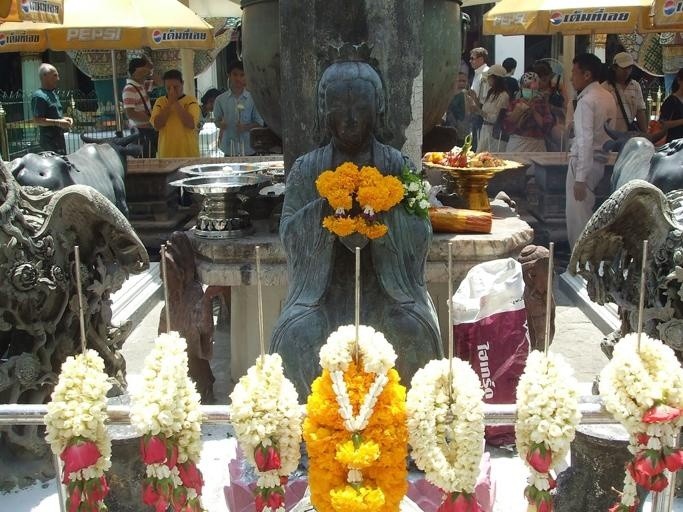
<box><xmin>523</xmin><ymin>88</ymin><xmax>539</xmax><ymax>100</ymax></box>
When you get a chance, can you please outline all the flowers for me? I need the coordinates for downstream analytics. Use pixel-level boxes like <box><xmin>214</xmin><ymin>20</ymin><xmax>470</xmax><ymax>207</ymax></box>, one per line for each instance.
<box><xmin>128</xmin><ymin>332</ymin><xmax>203</xmax><ymax>512</ymax></box>
<box><xmin>598</xmin><ymin>332</ymin><xmax>683</xmax><ymax>512</ymax></box>
<box><xmin>236</xmin><ymin>101</ymin><xmax>245</xmax><ymax>124</ymax></box>
<box><xmin>315</xmin><ymin>162</ymin><xmax>406</xmax><ymax>239</ymax></box>
<box><xmin>406</xmin><ymin>357</ymin><xmax>486</xmax><ymax>512</ymax></box>
<box><xmin>303</xmin><ymin>324</ymin><xmax>409</xmax><ymax>512</ymax></box>
<box><xmin>229</xmin><ymin>354</ymin><xmax>302</xmax><ymax>512</ymax></box>
<box><xmin>514</xmin><ymin>349</ymin><xmax>577</xmax><ymax>512</ymax></box>
<box><xmin>44</xmin><ymin>349</ymin><xmax>112</xmax><ymax>512</ymax></box>
<box><xmin>396</xmin><ymin>162</ymin><xmax>432</xmax><ymax>219</ymax></box>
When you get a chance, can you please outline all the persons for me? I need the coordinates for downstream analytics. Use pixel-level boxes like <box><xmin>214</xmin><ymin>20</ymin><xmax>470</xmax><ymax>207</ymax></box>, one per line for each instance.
<box><xmin>31</xmin><ymin>63</ymin><xmax>73</xmax><ymax>155</ymax></box>
<box><xmin>268</xmin><ymin>43</ymin><xmax>444</xmax><ymax>406</ymax></box>
<box><xmin>121</xmin><ymin>57</ymin><xmax>264</xmax><ymax>159</ymax></box>
<box><xmin>446</xmin><ymin>47</ymin><xmax>566</xmax><ymax>152</ymax></box>
<box><xmin>564</xmin><ymin>52</ymin><xmax>617</xmax><ymax>252</ymax></box>
<box><xmin>658</xmin><ymin>68</ymin><xmax>683</xmax><ymax>144</ymax></box>
<box><xmin>598</xmin><ymin>52</ymin><xmax>648</xmax><ymax>135</ymax></box>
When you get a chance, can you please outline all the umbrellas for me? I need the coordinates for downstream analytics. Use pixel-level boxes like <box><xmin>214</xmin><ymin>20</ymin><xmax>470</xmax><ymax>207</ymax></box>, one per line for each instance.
<box><xmin>650</xmin><ymin>0</ymin><xmax>682</xmax><ymax>30</ymax></box>
<box><xmin>483</xmin><ymin>0</ymin><xmax>683</xmax><ymax>35</ymax></box>
<box><xmin>178</xmin><ymin>0</ymin><xmax>244</xmax><ymax>45</ymax></box>
<box><xmin>0</xmin><ymin>1</ymin><xmax>214</xmax><ymax>136</ymax></box>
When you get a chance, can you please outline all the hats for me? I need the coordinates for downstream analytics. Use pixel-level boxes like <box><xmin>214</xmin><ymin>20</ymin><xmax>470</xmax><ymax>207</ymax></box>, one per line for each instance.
<box><xmin>612</xmin><ymin>52</ymin><xmax>634</xmax><ymax>68</ymax></box>
<box><xmin>482</xmin><ymin>65</ymin><xmax>515</xmax><ymax>78</ymax></box>
<box><xmin>521</xmin><ymin>72</ymin><xmax>540</xmax><ymax>85</ymax></box>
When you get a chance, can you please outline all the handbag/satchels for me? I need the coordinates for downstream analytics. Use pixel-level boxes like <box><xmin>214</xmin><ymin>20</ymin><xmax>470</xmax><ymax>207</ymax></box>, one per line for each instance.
<box><xmin>629</xmin><ymin>121</ymin><xmax>641</xmax><ymax>132</ymax></box>
